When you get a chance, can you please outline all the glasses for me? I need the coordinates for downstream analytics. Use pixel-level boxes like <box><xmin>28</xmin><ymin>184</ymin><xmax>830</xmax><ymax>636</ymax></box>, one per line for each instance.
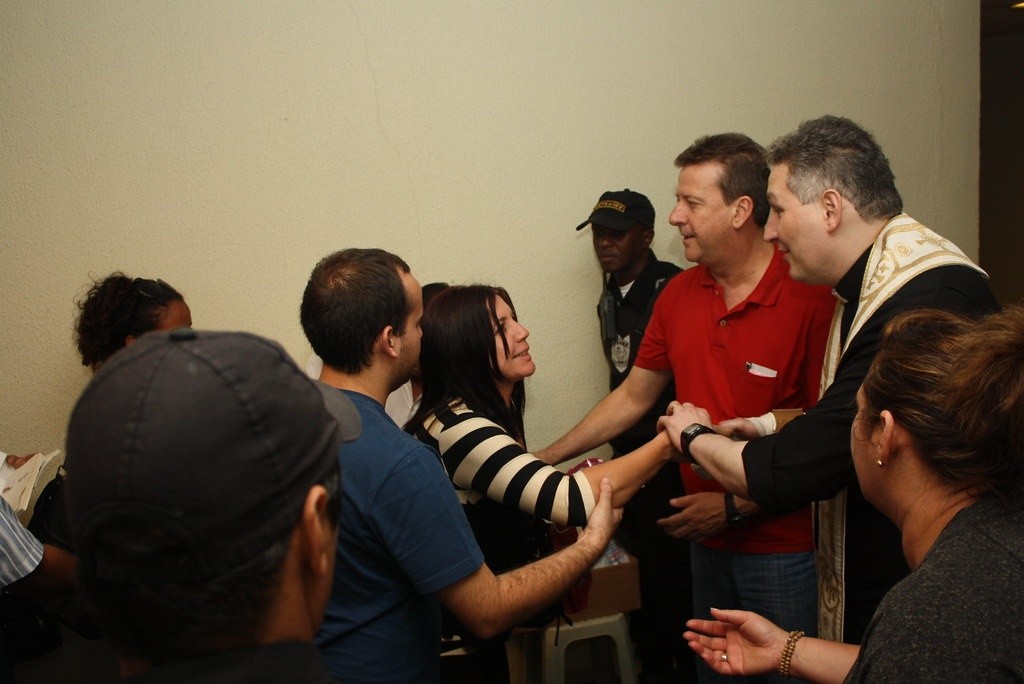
<box><xmin>102</xmin><ymin>277</ymin><xmax>177</xmax><ymax>329</ymax></box>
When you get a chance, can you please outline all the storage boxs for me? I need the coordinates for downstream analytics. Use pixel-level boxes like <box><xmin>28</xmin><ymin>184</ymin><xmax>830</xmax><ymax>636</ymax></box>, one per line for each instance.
<box><xmin>512</xmin><ymin>548</ymin><xmax>641</xmax><ymax>635</ymax></box>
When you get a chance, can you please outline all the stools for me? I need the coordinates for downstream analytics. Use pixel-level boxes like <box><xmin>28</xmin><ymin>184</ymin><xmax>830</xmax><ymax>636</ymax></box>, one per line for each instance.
<box><xmin>505</xmin><ymin>614</ymin><xmax>639</xmax><ymax>684</ymax></box>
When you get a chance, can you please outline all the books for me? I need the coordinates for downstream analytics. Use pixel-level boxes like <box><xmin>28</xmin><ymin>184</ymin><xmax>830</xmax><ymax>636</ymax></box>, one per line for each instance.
<box><xmin>0</xmin><ymin>449</ymin><xmax>61</xmax><ymax>517</ymax></box>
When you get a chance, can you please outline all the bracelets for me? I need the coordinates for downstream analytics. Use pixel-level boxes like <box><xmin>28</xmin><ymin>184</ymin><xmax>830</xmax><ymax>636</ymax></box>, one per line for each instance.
<box><xmin>780</xmin><ymin>631</ymin><xmax>805</xmax><ymax>675</ymax></box>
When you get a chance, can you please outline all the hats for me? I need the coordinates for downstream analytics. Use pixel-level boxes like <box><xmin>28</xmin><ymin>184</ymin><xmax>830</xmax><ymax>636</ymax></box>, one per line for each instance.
<box><xmin>576</xmin><ymin>189</ymin><xmax>656</xmax><ymax>232</ymax></box>
<box><xmin>61</xmin><ymin>329</ymin><xmax>363</xmax><ymax>590</ymax></box>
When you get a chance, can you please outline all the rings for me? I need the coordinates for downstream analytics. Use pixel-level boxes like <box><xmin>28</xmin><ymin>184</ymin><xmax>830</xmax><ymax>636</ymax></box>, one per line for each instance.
<box><xmin>722</xmin><ymin>653</ymin><xmax>727</xmax><ymax>661</ymax></box>
<box><xmin>671</xmin><ymin>403</ymin><xmax>681</xmax><ymax>411</ymax></box>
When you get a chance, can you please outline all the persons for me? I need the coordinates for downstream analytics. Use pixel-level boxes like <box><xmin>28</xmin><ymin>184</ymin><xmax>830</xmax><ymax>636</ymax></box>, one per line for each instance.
<box><xmin>576</xmin><ymin>188</ymin><xmax>694</xmax><ymax>684</ymax></box>
<box><xmin>66</xmin><ymin>329</ymin><xmax>343</xmax><ymax>684</ymax></box>
<box><xmin>656</xmin><ymin>110</ymin><xmax>1002</xmax><ymax>644</ymax></box>
<box><xmin>0</xmin><ymin>451</ymin><xmax>80</xmax><ymax>592</ymax></box>
<box><xmin>306</xmin><ymin>283</ymin><xmax>450</xmax><ymax>430</ymax></box>
<box><xmin>401</xmin><ymin>284</ymin><xmax>678</xmax><ymax>684</ymax></box>
<box><xmin>532</xmin><ymin>131</ymin><xmax>838</xmax><ymax>684</ymax></box>
<box><xmin>684</xmin><ymin>310</ymin><xmax>1024</xmax><ymax>684</ymax></box>
<box><xmin>75</xmin><ymin>276</ymin><xmax>193</xmax><ymax>371</ymax></box>
<box><xmin>299</xmin><ymin>250</ymin><xmax>624</xmax><ymax>684</ymax></box>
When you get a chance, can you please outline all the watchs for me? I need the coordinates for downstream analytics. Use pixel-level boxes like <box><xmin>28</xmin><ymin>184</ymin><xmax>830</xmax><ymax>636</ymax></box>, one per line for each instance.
<box><xmin>724</xmin><ymin>493</ymin><xmax>744</xmax><ymax>526</ymax></box>
<box><xmin>680</xmin><ymin>423</ymin><xmax>717</xmax><ymax>460</ymax></box>
